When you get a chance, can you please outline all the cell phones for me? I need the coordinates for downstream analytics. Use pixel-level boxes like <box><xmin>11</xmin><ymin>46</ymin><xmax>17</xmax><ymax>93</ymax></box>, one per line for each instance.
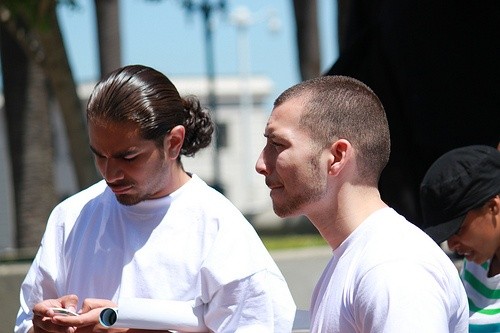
<box><xmin>51</xmin><ymin>308</ymin><xmax>78</xmax><ymax>317</ymax></box>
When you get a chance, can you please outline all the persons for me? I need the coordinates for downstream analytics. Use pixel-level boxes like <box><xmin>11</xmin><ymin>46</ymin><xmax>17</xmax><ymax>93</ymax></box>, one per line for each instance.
<box><xmin>13</xmin><ymin>63</ymin><xmax>297</xmax><ymax>333</ymax></box>
<box><xmin>255</xmin><ymin>75</ymin><xmax>469</xmax><ymax>333</ymax></box>
<box><xmin>416</xmin><ymin>144</ymin><xmax>500</xmax><ymax>333</ymax></box>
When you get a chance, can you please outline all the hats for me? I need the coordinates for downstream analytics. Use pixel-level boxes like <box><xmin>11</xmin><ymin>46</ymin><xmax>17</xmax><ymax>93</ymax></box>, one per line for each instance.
<box><xmin>418</xmin><ymin>144</ymin><xmax>500</xmax><ymax>244</ymax></box>
